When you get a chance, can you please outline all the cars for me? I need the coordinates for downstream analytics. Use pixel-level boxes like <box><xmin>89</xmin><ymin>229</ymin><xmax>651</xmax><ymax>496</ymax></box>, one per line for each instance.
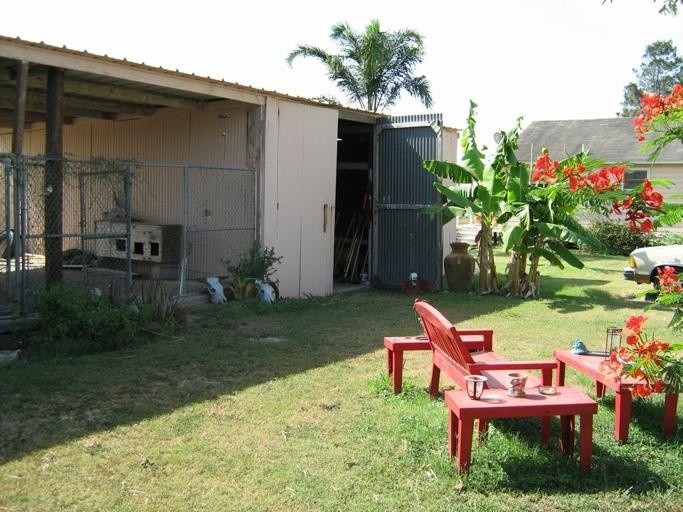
<box><xmin>620</xmin><ymin>240</ymin><xmax>682</xmax><ymax>293</ymax></box>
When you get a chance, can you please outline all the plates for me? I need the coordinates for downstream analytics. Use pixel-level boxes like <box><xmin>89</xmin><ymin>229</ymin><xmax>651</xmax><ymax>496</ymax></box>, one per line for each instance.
<box><xmin>505</xmin><ymin>392</ymin><xmax>527</xmax><ymax>397</ymax></box>
<box><xmin>572</xmin><ymin>350</ymin><xmax>586</xmax><ymax>355</ymax></box>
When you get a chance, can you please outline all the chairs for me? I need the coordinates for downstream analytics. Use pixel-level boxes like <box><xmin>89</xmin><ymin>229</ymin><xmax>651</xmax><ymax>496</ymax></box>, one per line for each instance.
<box><xmin>413</xmin><ymin>299</ymin><xmax>557</xmax><ymax>440</ymax></box>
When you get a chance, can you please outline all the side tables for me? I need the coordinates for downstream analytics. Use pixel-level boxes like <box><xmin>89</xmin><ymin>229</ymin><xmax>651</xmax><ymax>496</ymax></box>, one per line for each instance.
<box><xmin>384</xmin><ymin>334</ymin><xmax>485</xmax><ymax>394</ymax></box>
<box><xmin>442</xmin><ymin>385</ymin><xmax>598</xmax><ymax>473</ymax></box>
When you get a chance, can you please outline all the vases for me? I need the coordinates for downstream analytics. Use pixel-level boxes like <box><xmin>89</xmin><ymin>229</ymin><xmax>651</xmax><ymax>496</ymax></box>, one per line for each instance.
<box><xmin>443</xmin><ymin>242</ymin><xmax>474</xmax><ymax>293</ymax></box>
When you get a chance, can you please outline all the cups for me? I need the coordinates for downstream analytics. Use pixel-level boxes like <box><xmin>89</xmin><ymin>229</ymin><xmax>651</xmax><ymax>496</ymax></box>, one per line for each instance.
<box><xmin>505</xmin><ymin>372</ymin><xmax>528</xmax><ymax>396</ymax></box>
<box><xmin>574</xmin><ymin>339</ymin><xmax>585</xmax><ymax>351</ymax></box>
<box><xmin>464</xmin><ymin>374</ymin><xmax>488</xmax><ymax>401</ymax></box>
